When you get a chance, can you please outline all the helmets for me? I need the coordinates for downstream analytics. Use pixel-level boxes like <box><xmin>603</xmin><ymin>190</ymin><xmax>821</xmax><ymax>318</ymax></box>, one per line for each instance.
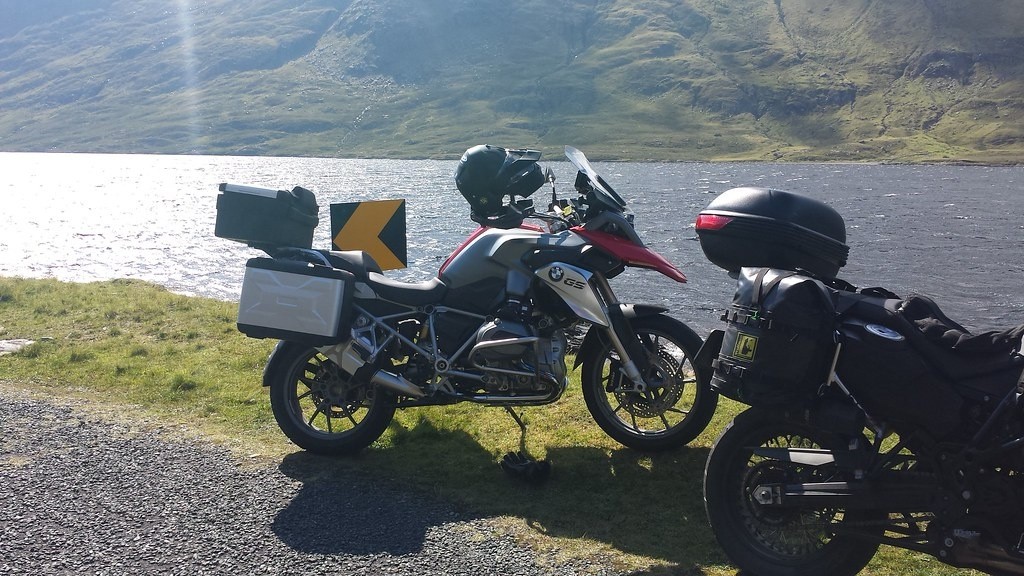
<box><xmin>454</xmin><ymin>146</ymin><xmax>549</xmax><ymax>228</ymax></box>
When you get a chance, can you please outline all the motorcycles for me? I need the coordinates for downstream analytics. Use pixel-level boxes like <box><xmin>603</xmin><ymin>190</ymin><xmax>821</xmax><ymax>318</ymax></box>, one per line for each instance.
<box><xmin>211</xmin><ymin>145</ymin><xmax>722</xmax><ymax>454</ymax></box>
<box><xmin>695</xmin><ymin>186</ymin><xmax>1024</xmax><ymax>576</ymax></box>
